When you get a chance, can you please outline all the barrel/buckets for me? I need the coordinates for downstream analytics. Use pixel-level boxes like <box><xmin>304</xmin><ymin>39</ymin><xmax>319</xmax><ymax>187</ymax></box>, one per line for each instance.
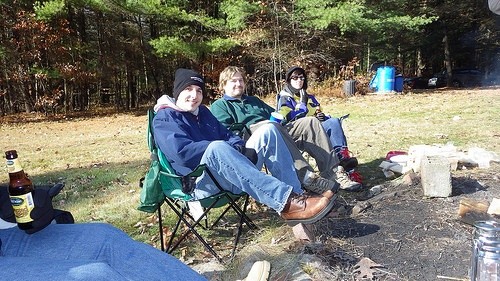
<box><xmin>395</xmin><ymin>75</ymin><xmax>403</xmax><ymax>91</ymax></box>
<box><xmin>377</xmin><ymin>66</ymin><xmax>394</xmax><ymax>91</ymax></box>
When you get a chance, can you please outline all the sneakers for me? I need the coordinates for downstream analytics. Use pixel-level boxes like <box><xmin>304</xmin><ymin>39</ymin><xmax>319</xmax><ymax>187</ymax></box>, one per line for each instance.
<box><xmin>347</xmin><ymin>169</ymin><xmax>363</xmax><ymax>185</ymax></box>
<box><xmin>323</xmin><ymin>166</ymin><xmax>363</xmax><ymax>192</ymax></box>
<box><xmin>306</xmin><ymin>176</ymin><xmax>341</xmax><ymax>195</ymax></box>
<box><xmin>334</xmin><ymin>146</ymin><xmax>358</xmax><ymax>171</ymax></box>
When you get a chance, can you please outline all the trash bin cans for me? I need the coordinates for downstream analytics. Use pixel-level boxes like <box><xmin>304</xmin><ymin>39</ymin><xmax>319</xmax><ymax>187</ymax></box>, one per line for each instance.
<box><xmin>343</xmin><ymin>80</ymin><xmax>356</xmax><ymax>96</ymax></box>
<box><xmin>377</xmin><ymin>66</ymin><xmax>396</xmax><ymax>92</ymax></box>
<box><xmin>395</xmin><ymin>74</ymin><xmax>403</xmax><ymax>92</ymax></box>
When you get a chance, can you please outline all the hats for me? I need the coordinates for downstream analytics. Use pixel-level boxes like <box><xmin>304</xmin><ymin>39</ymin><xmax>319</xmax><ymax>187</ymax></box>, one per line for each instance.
<box><xmin>173</xmin><ymin>68</ymin><xmax>205</xmax><ymax>104</ymax></box>
<box><xmin>286</xmin><ymin>66</ymin><xmax>308</xmax><ymax>95</ymax></box>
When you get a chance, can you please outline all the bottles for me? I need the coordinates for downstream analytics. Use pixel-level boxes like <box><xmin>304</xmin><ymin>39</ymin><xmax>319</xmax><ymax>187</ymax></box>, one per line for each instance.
<box><xmin>472</xmin><ymin>220</ymin><xmax>500</xmax><ymax>281</ymax></box>
<box><xmin>6</xmin><ymin>150</ymin><xmax>35</xmax><ymax>230</ymax></box>
<box><xmin>316</xmin><ymin>104</ymin><xmax>320</xmax><ymax>115</ymax></box>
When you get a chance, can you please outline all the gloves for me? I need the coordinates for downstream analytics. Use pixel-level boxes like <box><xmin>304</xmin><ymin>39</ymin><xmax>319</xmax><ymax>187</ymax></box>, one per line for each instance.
<box><xmin>0</xmin><ymin>186</ymin><xmax>55</xmax><ymax>234</ymax></box>
<box><xmin>300</xmin><ymin>89</ymin><xmax>308</xmax><ymax>105</ymax></box>
<box><xmin>242</xmin><ymin>148</ymin><xmax>258</xmax><ymax>165</ymax></box>
<box><xmin>317</xmin><ymin>113</ymin><xmax>325</xmax><ymax>122</ymax></box>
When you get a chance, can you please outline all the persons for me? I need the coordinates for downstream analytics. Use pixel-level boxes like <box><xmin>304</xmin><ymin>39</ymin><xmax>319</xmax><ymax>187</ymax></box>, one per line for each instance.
<box><xmin>277</xmin><ymin>65</ymin><xmax>362</xmax><ymax>183</ymax></box>
<box><xmin>210</xmin><ymin>66</ymin><xmax>362</xmax><ymax>195</ymax></box>
<box><xmin>0</xmin><ymin>184</ymin><xmax>271</xmax><ymax>281</ymax></box>
<box><xmin>152</xmin><ymin>68</ymin><xmax>337</xmax><ymax>227</ymax></box>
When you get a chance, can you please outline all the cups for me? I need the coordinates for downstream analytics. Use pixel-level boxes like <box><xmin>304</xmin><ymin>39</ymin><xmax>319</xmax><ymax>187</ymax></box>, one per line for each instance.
<box><xmin>270</xmin><ymin>112</ymin><xmax>284</xmax><ymax>123</ymax></box>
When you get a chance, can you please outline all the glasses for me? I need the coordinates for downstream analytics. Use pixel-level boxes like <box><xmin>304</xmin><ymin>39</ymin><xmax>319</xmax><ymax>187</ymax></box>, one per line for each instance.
<box><xmin>290</xmin><ymin>75</ymin><xmax>306</xmax><ymax>80</ymax></box>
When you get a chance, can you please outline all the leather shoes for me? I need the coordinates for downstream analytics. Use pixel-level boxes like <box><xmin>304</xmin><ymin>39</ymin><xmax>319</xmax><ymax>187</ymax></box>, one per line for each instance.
<box><xmin>292</xmin><ymin>190</ymin><xmax>338</xmax><ymax>203</ymax></box>
<box><xmin>281</xmin><ymin>192</ymin><xmax>334</xmax><ymax>228</ymax></box>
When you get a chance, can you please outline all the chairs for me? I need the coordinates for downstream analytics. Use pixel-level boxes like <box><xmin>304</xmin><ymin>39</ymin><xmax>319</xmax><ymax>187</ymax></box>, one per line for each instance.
<box><xmin>139</xmin><ymin>109</ymin><xmax>251</xmax><ymax>267</ymax></box>
